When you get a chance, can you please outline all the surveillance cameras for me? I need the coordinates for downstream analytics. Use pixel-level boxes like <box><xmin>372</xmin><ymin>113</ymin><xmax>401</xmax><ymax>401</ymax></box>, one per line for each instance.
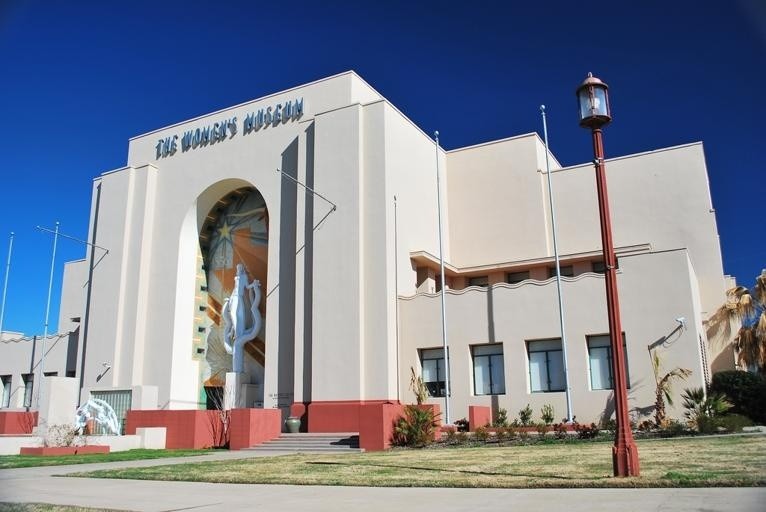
<box><xmin>676</xmin><ymin>317</ymin><xmax>684</xmax><ymax>322</ymax></box>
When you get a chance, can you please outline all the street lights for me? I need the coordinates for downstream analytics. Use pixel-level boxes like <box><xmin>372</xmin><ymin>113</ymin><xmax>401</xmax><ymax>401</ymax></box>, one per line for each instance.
<box><xmin>575</xmin><ymin>70</ymin><xmax>641</xmax><ymax>477</ymax></box>
